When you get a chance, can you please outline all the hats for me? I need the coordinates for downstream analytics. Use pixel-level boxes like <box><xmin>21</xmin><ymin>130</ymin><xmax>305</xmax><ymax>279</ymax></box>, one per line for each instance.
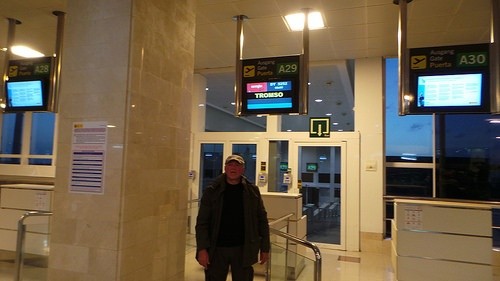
<box><xmin>225</xmin><ymin>155</ymin><xmax>245</xmax><ymax>165</ymax></box>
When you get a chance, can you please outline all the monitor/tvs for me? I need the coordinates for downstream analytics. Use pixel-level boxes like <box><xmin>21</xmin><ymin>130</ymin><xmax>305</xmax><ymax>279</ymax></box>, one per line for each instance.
<box><xmin>415</xmin><ymin>72</ymin><xmax>486</xmax><ymax>111</ymax></box>
<box><xmin>243</xmin><ymin>78</ymin><xmax>295</xmax><ymax>113</ymax></box>
<box><xmin>5</xmin><ymin>79</ymin><xmax>45</xmax><ymax>112</ymax></box>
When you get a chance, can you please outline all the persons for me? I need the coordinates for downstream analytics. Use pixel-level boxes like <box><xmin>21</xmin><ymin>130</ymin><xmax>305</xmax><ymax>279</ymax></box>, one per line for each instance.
<box><xmin>195</xmin><ymin>155</ymin><xmax>271</xmax><ymax>281</ymax></box>
